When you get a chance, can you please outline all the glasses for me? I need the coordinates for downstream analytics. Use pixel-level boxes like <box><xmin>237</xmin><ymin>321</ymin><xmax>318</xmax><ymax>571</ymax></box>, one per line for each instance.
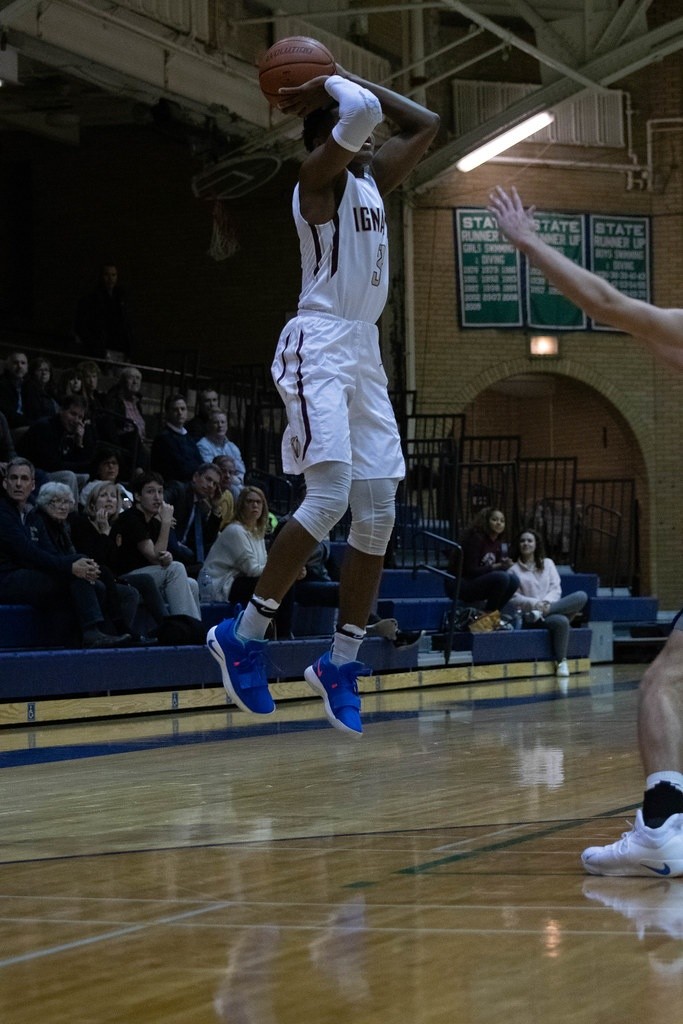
<box><xmin>50</xmin><ymin>499</ymin><xmax>73</xmax><ymax>507</ymax></box>
<box><xmin>245</xmin><ymin>497</ymin><xmax>263</xmax><ymax>504</ymax></box>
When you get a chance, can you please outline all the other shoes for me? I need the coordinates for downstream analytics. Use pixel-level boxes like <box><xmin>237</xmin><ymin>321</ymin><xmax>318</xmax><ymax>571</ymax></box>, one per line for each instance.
<box><xmin>494</xmin><ymin>620</ymin><xmax>512</xmax><ymax>631</ymax></box>
<box><xmin>174</xmin><ymin>635</ymin><xmax>202</xmax><ymax>646</ymax></box>
<box><xmin>82</xmin><ymin>629</ymin><xmax>131</xmax><ymax>649</ymax></box>
<box><xmin>128</xmin><ymin>636</ymin><xmax>158</xmax><ymax>646</ymax></box>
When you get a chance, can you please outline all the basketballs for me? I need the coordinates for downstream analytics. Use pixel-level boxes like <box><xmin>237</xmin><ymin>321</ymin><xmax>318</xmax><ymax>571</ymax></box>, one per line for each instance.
<box><xmin>258</xmin><ymin>35</ymin><xmax>337</xmax><ymax>113</ymax></box>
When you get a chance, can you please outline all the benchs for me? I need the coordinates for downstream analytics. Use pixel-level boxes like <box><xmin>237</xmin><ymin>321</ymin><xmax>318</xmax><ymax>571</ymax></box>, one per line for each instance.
<box><xmin>429</xmin><ymin>627</ymin><xmax>593</xmax><ymax>667</ymax></box>
<box><xmin>580</xmin><ymin>597</ymin><xmax>660</xmax><ymax>623</ymax></box>
<box><xmin>560</xmin><ymin>574</ymin><xmax>598</xmax><ymax>597</ymax></box>
<box><xmin>0</xmin><ymin>602</ymin><xmax>339</xmax><ymax>648</ymax></box>
<box><xmin>376</xmin><ymin>598</ymin><xmax>453</xmax><ymax>631</ymax></box>
<box><xmin>378</xmin><ymin>569</ymin><xmax>448</xmax><ymax>599</ymax></box>
<box><xmin>398</xmin><ymin>522</ymin><xmax>450</xmax><ymax>550</ymax></box>
<box><xmin>338</xmin><ymin>505</ymin><xmax>419</xmax><ymax>525</ymax></box>
<box><xmin>0</xmin><ymin>632</ymin><xmax>419</xmax><ymax>701</ymax></box>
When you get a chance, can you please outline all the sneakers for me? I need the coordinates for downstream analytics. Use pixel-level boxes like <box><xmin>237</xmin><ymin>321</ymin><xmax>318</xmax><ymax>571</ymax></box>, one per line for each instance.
<box><xmin>206</xmin><ymin>611</ymin><xmax>276</xmax><ymax>716</ymax></box>
<box><xmin>581</xmin><ymin>875</ymin><xmax>683</xmax><ymax>941</ymax></box>
<box><xmin>581</xmin><ymin>810</ymin><xmax>682</xmax><ymax>878</ymax></box>
<box><xmin>305</xmin><ymin>644</ymin><xmax>372</xmax><ymax>739</ymax></box>
<box><xmin>525</xmin><ymin>610</ymin><xmax>545</xmax><ymax>623</ymax></box>
<box><xmin>555</xmin><ymin>658</ymin><xmax>569</xmax><ymax>676</ymax></box>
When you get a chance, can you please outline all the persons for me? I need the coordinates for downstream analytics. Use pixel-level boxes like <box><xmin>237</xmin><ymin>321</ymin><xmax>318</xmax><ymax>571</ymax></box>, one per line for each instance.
<box><xmin>89</xmin><ymin>262</ymin><xmax>135</xmax><ymax>363</ymax></box>
<box><xmin>486</xmin><ymin>185</ymin><xmax>683</xmax><ymax>878</ymax></box>
<box><xmin>463</xmin><ymin>507</ymin><xmax>588</xmax><ymax>676</ymax></box>
<box><xmin>0</xmin><ymin>350</ymin><xmax>425</xmax><ymax>652</ymax></box>
<box><xmin>205</xmin><ymin>63</ymin><xmax>440</xmax><ymax>741</ymax></box>
